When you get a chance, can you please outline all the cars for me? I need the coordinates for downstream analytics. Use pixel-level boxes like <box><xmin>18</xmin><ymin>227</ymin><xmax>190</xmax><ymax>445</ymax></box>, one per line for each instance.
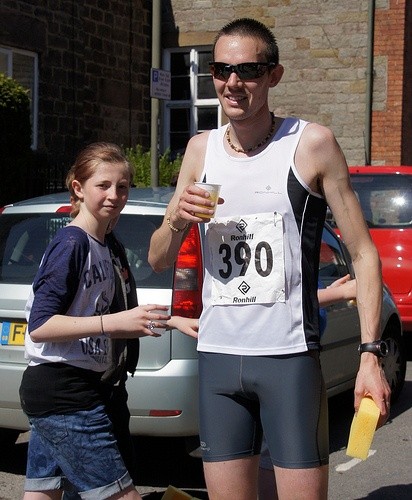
<box><xmin>0</xmin><ymin>186</ymin><xmax>406</xmax><ymax>467</ymax></box>
<box><xmin>327</xmin><ymin>166</ymin><xmax>412</xmax><ymax>331</ymax></box>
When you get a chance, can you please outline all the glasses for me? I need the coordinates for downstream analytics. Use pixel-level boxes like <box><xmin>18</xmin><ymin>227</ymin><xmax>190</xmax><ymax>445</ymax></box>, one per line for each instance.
<box><xmin>209</xmin><ymin>62</ymin><xmax>276</xmax><ymax>80</ymax></box>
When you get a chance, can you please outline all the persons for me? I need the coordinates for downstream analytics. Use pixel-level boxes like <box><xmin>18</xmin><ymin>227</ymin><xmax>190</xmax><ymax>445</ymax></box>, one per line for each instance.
<box><xmin>147</xmin><ymin>18</ymin><xmax>392</xmax><ymax>500</ymax></box>
<box><xmin>18</xmin><ymin>142</ymin><xmax>172</xmax><ymax>500</ymax></box>
<box><xmin>316</xmin><ymin>274</ymin><xmax>356</xmax><ymax>339</ymax></box>
<box><xmin>61</xmin><ymin>196</ymin><xmax>200</xmax><ymax>500</ymax></box>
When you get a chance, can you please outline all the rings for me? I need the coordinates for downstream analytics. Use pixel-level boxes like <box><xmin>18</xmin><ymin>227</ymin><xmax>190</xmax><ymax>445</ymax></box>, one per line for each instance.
<box><xmin>148</xmin><ymin>321</ymin><xmax>156</xmax><ymax>330</ymax></box>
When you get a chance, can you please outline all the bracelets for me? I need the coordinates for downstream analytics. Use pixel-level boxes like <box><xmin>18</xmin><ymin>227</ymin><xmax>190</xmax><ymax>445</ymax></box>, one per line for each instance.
<box><xmin>167</xmin><ymin>216</ymin><xmax>188</xmax><ymax>232</ymax></box>
<box><xmin>101</xmin><ymin>315</ymin><xmax>105</xmax><ymax>334</ymax></box>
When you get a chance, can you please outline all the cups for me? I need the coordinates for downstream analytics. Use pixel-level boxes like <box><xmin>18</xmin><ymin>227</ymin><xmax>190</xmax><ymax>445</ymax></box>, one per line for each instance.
<box><xmin>194</xmin><ymin>182</ymin><xmax>221</xmax><ymax>218</ymax></box>
<box><xmin>148</xmin><ymin>305</ymin><xmax>170</xmax><ymax>334</ymax></box>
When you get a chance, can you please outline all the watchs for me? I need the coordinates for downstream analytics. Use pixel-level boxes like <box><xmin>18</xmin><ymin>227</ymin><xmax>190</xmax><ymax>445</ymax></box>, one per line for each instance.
<box><xmin>358</xmin><ymin>339</ymin><xmax>389</xmax><ymax>359</ymax></box>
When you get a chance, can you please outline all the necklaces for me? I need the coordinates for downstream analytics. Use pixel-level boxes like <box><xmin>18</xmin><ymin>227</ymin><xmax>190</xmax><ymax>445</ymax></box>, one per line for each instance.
<box><xmin>226</xmin><ymin>110</ymin><xmax>276</xmax><ymax>153</ymax></box>
